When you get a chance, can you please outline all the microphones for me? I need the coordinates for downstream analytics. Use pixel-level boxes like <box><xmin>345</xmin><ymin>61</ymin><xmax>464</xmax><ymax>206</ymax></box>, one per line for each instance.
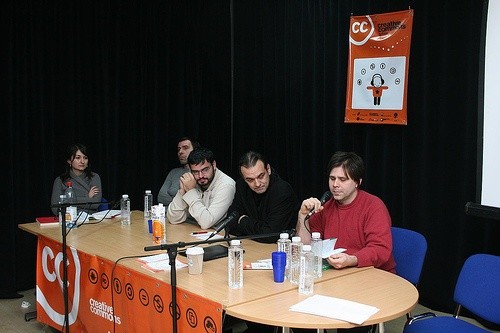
<box><xmin>210</xmin><ymin>211</ymin><xmax>239</xmax><ymax>237</ymax></box>
<box><xmin>303</xmin><ymin>192</ymin><xmax>332</xmax><ymax>222</ymax></box>
<box><xmin>112</xmin><ymin>200</ymin><xmax>121</xmax><ymax>209</ymax></box>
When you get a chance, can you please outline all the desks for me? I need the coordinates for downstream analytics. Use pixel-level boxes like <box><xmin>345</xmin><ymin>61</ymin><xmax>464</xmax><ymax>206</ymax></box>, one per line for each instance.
<box><xmin>18</xmin><ymin>210</ymin><xmax>420</xmax><ymax>333</ymax></box>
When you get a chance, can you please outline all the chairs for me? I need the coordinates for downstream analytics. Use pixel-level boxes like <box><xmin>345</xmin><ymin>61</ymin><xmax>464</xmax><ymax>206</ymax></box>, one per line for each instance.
<box><xmin>389</xmin><ymin>225</ymin><xmax>500</xmax><ymax>333</ymax></box>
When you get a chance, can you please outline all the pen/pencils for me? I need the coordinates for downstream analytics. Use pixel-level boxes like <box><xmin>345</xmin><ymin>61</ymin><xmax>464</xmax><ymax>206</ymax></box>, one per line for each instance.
<box><xmin>193</xmin><ymin>231</ymin><xmax>208</xmax><ymax>234</ymax></box>
<box><xmin>244</xmin><ymin>265</ymin><xmax>252</xmax><ymax>268</ymax></box>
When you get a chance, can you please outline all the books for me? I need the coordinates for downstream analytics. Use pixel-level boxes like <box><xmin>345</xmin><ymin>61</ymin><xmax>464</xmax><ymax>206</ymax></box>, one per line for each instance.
<box><xmin>310</xmin><ymin>238</ymin><xmax>347</xmax><ymax>271</ymax></box>
<box><xmin>35</xmin><ymin>216</ymin><xmax>59</xmax><ymax>225</ymax></box>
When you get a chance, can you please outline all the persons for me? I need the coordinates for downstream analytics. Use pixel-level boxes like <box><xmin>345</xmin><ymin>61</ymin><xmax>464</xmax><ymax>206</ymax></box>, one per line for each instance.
<box><xmin>226</xmin><ymin>151</ymin><xmax>301</xmax><ymax>333</ymax></box>
<box><xmin>167</xmin><ymin>147</ymin><xmax>236</xmax><ymax>230</ymax></box>
<box><xmin>157</xmin><ymin>136</ymin><xmax>200</xmax><ymax>206</ymax></box>
<box><xmin>51</xmin><ymin>143</ymin><xmax>102</xmax><ymax>217</ymax></box>
<box><xmin>295</xmin><ymin>151</ymin><xmax>397</xmax><ymax>333</ymax></box>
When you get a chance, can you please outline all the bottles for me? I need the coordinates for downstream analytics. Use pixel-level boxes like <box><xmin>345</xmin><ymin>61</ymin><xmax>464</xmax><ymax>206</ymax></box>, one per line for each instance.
<box><xmin>144</xmin><ymin>190</ymin><xmax>152</xmax><ymax>219</ymax></box>
<box><xmin>311</xmin><ymin>233</ymin><xmax>323</xmax><ymax>278</ymax></box>
<box><xmin>63</xmin><ymin>182</ymin><xmax>78</xmax><ymax>228</ymax></box>
<box><xmin>58</xmin><ymin>195</ymin><xmax>63</xmax><ymax>225</ymax></box>
<box><xmin>290</xmin><ymin>237</ymin><xmax>303</xmax><ymax>283</ymax></box>
<box><xmin>278</xmin><ymin>233</ymin><xmax>291</xmax><ymax>279</ymax></box>
<box><xmin>120</xmin><ymin>195</ymin><xmax>130</xmax><ymax>225</ymax></box>
<box><xmin>227</xmin><ymin>240</ymin><xmax>244</xmax><ymax>290</ymax></box>
<box><xmin>298</xmin><ymin>245</ymin><xmax>313</xmax><ymax>295</ymax></box>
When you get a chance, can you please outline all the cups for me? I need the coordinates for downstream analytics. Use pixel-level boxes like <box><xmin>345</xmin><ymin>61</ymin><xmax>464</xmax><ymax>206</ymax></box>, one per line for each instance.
<box><xmin>186</xmin><ymin>247</ymin><xmax>204</xmax><ymax>274</ymax></box>
<box><xmin>272</xmin><ymin>252</ymin><xmax>286</xmax><ymax>283</ymax></box>
<box><xmin>148</xmin><ymin>220</ymin><xmax>152</xmax><ymax>234</ymax></box>
